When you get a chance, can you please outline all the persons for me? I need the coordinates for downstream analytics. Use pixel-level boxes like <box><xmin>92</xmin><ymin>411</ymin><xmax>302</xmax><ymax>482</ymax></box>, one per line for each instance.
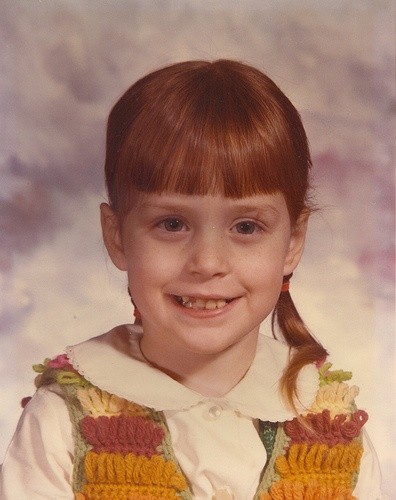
<box><xmin>1</xmin><ymin>57</ymin><xmax>386</xmax><ymax>499</ymax></box>
